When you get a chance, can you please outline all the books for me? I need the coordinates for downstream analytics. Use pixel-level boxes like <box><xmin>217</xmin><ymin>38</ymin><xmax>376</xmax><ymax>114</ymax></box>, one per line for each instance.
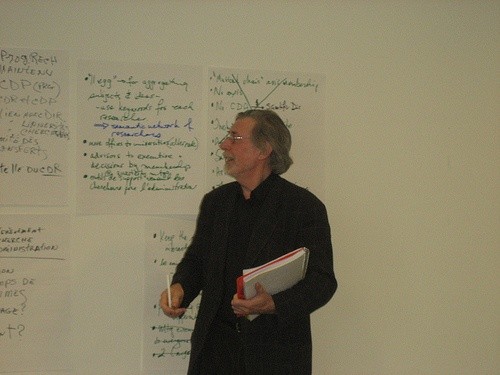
<box><xmin>235</xmin><ymin>247</ymin><xmax>310</xmax><ymax>323</ymax></box>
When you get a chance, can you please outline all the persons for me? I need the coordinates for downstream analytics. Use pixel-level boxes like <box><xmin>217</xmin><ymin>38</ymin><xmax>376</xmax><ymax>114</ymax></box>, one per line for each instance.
<box><xmin>158</xmin><ymin>107</ymin><xmax>339</xmax><ymax>375</ymax></box>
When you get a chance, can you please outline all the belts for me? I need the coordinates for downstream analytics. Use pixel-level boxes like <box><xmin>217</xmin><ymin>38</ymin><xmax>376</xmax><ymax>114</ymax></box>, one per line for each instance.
<box><xmin>217</xmin><ymin>314</ymin><xmax>241</xmax><ymax>333</ymax></box>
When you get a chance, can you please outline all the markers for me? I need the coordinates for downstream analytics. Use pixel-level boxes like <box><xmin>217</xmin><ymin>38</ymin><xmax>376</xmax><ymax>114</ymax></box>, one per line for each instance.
<box><xmin>166</xmin><ymin>274</ymin><xmax>173</xmax><ymax>308</ymax></box>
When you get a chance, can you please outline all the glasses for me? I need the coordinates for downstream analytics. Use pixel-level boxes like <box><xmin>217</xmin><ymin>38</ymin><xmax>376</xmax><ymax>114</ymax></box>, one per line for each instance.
<box><xmin>226</xmin><ymin>134</ymin><xmax>251</xmax><ymax>145</ymax></box>
